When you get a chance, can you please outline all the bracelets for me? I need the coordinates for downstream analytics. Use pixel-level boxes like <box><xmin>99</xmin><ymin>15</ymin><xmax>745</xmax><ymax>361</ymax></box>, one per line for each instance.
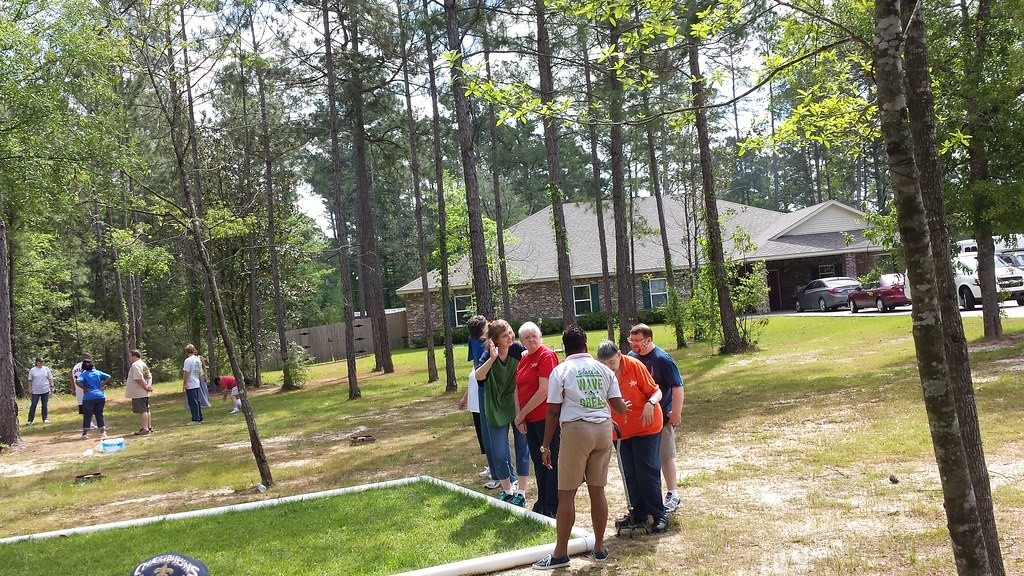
<box><xmin>540</xmin><ymin>446</ymin><xmax>549</xmax><ymax>453</ymax></box>
<box><xmin>49</xmin><ymin>385</ymin><xmax>54</xmax><ymax>388</ymax></box>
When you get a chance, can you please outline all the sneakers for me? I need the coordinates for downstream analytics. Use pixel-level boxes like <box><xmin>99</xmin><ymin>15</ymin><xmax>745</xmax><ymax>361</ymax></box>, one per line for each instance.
<box><xmin>479</xmin><ymin>466</ymin><xmax>492</xmax><ymax>478</ymax></box>
<box><xmin>495</xmin><ymin>490</ymin><xmax>513</xmax><ymax>502</ymax></box>
<box><xmin>483</xmin><ymin>479</ymin><xmax>501</xmax><ymax>489</ymax></box>
<box><xmin>592</xmin><ymin>547</ymin><xmax>609</xmax><ymax>561</ymax></box>
<box><xmin>664</xmin><ymin>491</ymin><xmax>683</xmax><ymax>512</ymax></box>
<box><xmin>509</xmin><ymin>493</ymin><xmax>527</xmax><ymax>508</ymax></box>
<box><xmin>510</xmin><ymin>480</ymin><xmax>518</xmax><ymax>488</ymax></box>
<box><xmin>531</xmin><ymin>554</ymin><xmax>570</xmax><ymax>568</ymax></box>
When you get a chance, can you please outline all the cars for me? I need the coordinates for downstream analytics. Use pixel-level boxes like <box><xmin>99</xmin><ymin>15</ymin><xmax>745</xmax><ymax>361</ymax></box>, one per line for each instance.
<box><xmin>847</xmin><ymin>273</ymin><xmax>912</xmax><ymax>313</ymax></box>
<box><xmin>793</xmin><ymin>277</ymin><xmax>861</xmax><ymax>312</ymax></box>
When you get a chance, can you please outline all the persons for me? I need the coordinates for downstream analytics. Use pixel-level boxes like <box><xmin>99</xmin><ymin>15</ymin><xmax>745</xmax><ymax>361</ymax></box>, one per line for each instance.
<box><xmin>513</xmin><ymin>321</ymin><xmax>559</xmax><ymax>517</ymax></box>
<box><xmin>597</xmin><ymin>339</ymin><xmax>669</xmax><ymax>531</ymax></box>
<box><xmin>468</xmin><ymin>314</ymin><xmax>503</xmax><ymax>489</ymax></box>
<box><xmin>72</xmin><ymin>352</ymin><xmax>98</xmax><ymax>430</ymax></box>
<box><xmin>186</xmin><ymin>349</ymin><xmax>211</xmax><ymax>409</ymax></box>
<box><xmin>182</xmin><ymin>343</ymin><xmax>205</xmax><ymax>426</ymax></box>
<box><xmin>213</xmin><ymin>375</ymin><xmax>245</xmax><ymax>413</ymax></box>
<box><xmin>125</xmin><ymin>350</ymin><xmax>155</xmax><ymax>434</ymax></box>
<box><xmin>627</xmin><ymin>323</ymin><xmax>685</xmax><ymax>512</ymax></box>
<box><xmin>475</xmin><ymin>318</ymin><xmax>530</xmax><ymax>507</ymax></box>
<box><xmin>532</xmin><ymin>323</ymin><xmax>633</xmax><ymax>570</ymax></box>
<box><xmin>76</xmin><ymin>359</ymin><xmax>111</xmax><ymax>437</ymax></box>
<box><xmin>26</xmin><ymin>357</ymin><xmax>54</xmax><ymax>426</ymax></box>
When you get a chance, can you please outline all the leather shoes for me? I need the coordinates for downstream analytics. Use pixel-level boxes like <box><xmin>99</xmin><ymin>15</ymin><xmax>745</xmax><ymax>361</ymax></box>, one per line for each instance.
<box><xmin>652</xmin><ymin>513</ymin><xmax>668</xmax><ymax>534</ymax></box>
<box><xmin>614</xmin><ymin>515</ymin><xmax>647</xmax><ymax>528</ymax></box>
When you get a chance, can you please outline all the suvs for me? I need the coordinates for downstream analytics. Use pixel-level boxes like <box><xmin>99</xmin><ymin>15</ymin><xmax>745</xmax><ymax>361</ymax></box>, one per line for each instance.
<box><xmin>904</xmin><ymin>252</ymin><xmax>1024</xmax><ymax>311</ymax></box>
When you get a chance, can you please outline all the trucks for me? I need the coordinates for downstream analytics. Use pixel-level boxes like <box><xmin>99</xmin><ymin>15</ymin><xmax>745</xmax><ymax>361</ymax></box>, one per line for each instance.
<box><xmin>952</xmin><ymin>233</ymin><xmax>1024</xmax><ymax>252</ymax></box>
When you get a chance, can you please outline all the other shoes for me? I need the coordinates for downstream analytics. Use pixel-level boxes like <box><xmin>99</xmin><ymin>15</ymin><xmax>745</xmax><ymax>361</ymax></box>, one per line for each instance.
<box><xmin>186</xmin><ymin>419</ymin><xmax>203</xmax><ymax>426</ymax></box>
<box><xmin>229</xmin><ymin>409</ymin><xmax>239</xmax><ymax>414</ymax></box>
<box><xmin>26</xmin><ymin>421</ymin><xmax>32</xmax><ymax>426</ymax></box>
<box><xmin>134</xmin><ymin>427</ymin><xmax>150</xmax><ymax>435</ymax></box>
<box><xmin>149</xmin><ymin>427</ymin><xmax>153</xmax><ymax>432</ymax></box>
<box><xmin>43</xmin><ymin>420</ymin><xmax>51</xmax><ymax>423</ymax></box>
<box><xmin>77</xmin><ymin>421</ymin><xmax>109</xmax><ymax>440</ymax></box>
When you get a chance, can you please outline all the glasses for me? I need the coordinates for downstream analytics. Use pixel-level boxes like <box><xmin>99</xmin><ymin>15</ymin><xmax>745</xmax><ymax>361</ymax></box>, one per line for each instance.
<box><xmin>626</xmin><ymin>336</ymin><xmax>647</xmax><ymax>343</ymax></box>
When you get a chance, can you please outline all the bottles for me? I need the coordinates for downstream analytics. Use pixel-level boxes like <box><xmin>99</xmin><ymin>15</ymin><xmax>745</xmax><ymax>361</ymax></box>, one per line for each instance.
<box><xmin>256</xmin><ymin>483</ymin><xmax>266</xmax><ymax>492</ymax></box>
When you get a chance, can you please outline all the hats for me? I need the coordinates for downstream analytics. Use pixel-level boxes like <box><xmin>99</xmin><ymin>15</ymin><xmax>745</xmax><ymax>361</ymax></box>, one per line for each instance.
<box><xmin>35</xmin><ymin>357</ymin><xmax>46</xmax><ymax>362</ymax></box>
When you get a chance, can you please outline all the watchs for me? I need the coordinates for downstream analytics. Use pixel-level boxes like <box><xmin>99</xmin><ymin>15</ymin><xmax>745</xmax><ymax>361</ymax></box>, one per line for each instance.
<box><xmin>647</xmin><ymin>399</ymin><xmax>656</xmax><ymax>407</ymax></box>
